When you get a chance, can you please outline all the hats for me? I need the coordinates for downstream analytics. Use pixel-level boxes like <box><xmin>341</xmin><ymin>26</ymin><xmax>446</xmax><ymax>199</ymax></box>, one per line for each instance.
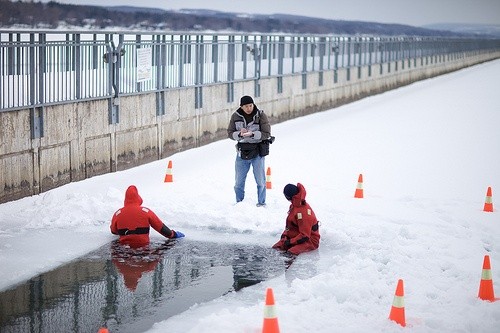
<box><xmin>284</xmin><ymin>183</ymin><xmax>299</xmax><ymax>200</ymax></box>
<box><xmin>240</xmin><ymin>95</ymin><xmax>254</xmax><ymax>106</ymax></box>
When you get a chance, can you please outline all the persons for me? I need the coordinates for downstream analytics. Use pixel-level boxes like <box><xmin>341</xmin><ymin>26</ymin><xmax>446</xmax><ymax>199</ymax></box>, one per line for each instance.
<box><xmin>272</xmin><ymin>184</ymin><xmax>320</xmax><ymax>256</ymax></box>
<box><xmin>110</xmin><ymin>185</ymin><xmax>185</xmax><ymax>248</ymax></box>
<box><xmin>227</xmin><ymin>96</ymin><xmax>271</xmax><ymax>207</ymax></box>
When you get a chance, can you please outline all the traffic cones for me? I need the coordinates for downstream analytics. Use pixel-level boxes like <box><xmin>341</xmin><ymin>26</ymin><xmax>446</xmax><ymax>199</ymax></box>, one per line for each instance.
<box><xmin>484</xmin><ymin>187</ymin><xmax>493</xmax><ymax>212</ymax></box>
<box><xmin>266</xmin><ymin>167</ymin><xmax>272</xmax><ymax>189</ymax></box>
<box><xmin>388</xmin><ymin>279</ymin><xmax>406</xmax><ymax>327</ymax></box>
<box><xmin>262</xmin><ymin>288</ymin><xmax>280</xmax><ymax>333</ymax></box>
<box><xmin>164</xmin><ymin>160</ymin><xmax>173</xmax><ymax>182</ymax></box>
<box><xmin>479</xmin><ymin>255</ymin><xmax>495</xmax><ymax>302</ymax></box>
<box><xmin>354</xmin><ymin>174</ymin><xmax>363</xmax><ymax>198</ymax></box>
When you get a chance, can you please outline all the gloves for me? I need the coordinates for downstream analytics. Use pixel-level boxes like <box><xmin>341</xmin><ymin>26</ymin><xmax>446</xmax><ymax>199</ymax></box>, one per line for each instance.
<box><xmin>171</xmin><ymin>230</ymin><xmax>185</xmax><ymax>238</ymax></box>
